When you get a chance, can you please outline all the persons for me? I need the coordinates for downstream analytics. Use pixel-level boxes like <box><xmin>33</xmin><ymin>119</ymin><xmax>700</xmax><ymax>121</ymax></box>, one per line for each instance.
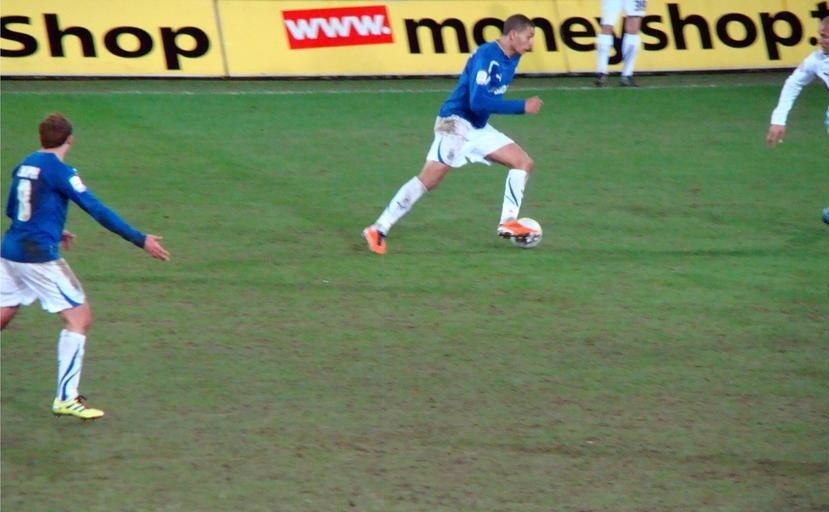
<box><xmin>591</xmin><ymin>0</ymin><xmax>650</xmax><ymax>87</ymax></box>
<box><xmin>766</xmin><ymin>16</ymin><xmax>829</xmax><ymax>226</ymax></box>
<box><xmin>0</xmin><ymin>112</ymin><xmax>171</xmax><ymax>421</ymax></box>
<box><xmin>361</xmin><ymin>15</ymin><xmax>544</xmax><ymax>255</ymax></box>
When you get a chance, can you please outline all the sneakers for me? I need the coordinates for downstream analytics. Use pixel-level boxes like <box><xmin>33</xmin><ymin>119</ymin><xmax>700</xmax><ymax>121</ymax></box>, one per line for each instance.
<box><xmin>496</xmin><ymin>218</ymin><xmax>542</xmax><ymax>243</ymax></box>
<box><xmin>593</xmin><ymin>74</ymin><xmax>640</xmax><ymax>88</ymax></box>
<box><xmin>52</xmin><ymin>398</ymin><xmax>104</xmax><ymax>421</ymax></box>
<box><xmin>362</xmin><ymin>226</ymin><xmax>387</xmax><ymax>255</ymax></box>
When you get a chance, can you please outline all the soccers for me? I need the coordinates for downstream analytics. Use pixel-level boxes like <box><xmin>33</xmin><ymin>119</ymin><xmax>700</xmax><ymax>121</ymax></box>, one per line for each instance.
<box><xmin>510</xmin><ymin>217</ymin><xmax>542</xmax><ymax>248</ymax></box>
